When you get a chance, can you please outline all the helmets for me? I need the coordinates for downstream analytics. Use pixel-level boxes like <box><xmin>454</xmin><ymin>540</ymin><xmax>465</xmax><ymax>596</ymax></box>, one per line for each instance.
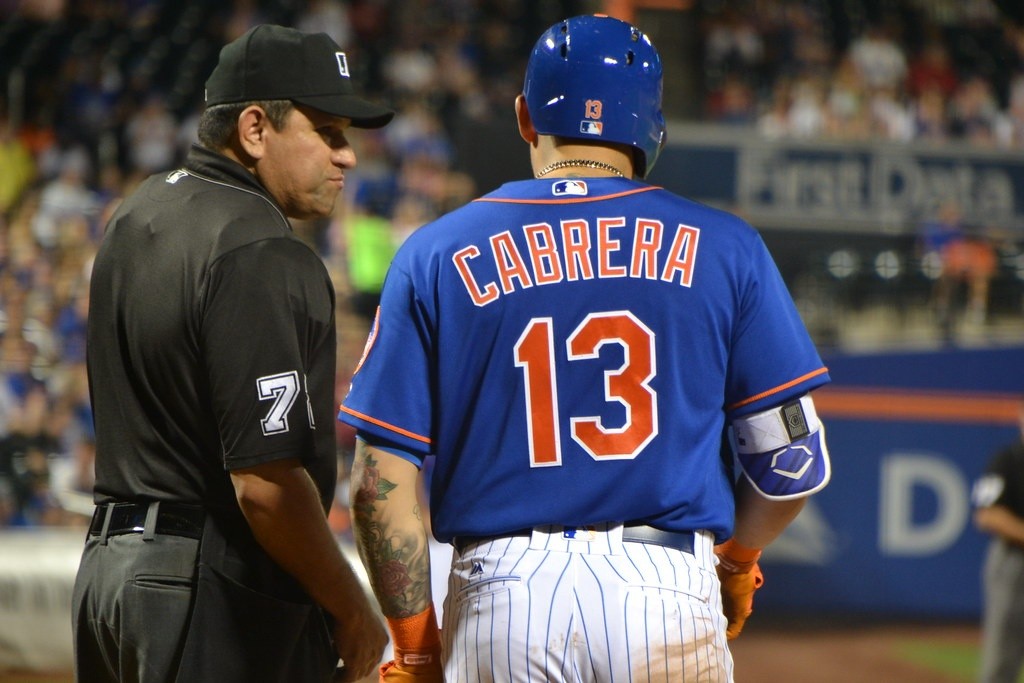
<box><xmin>524</xmin><ymin>13</ymin><xmax>668</xmax><ymax>181</ymax></box>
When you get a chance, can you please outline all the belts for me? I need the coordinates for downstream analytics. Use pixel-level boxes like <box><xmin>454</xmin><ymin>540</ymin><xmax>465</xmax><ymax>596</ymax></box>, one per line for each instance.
<box><xmin>90</xmin><ymin>504</ymin><xmax>206</xmax><ymax>540</ymax></box>
<box><xmin>620</xmin><ymin>521</ymin><xmax>695</xmax><ymax>555</ymax></box>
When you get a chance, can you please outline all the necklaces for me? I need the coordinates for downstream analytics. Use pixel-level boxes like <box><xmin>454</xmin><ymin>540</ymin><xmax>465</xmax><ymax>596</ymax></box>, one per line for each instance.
<box><xmin>534</xmin><ymin>159</ymin><xmax>626</xmax><ymax>180</ymax></box>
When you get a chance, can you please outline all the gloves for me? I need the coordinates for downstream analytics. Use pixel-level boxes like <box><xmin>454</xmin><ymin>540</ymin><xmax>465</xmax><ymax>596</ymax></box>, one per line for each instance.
<box><xmin>378</xmin><ymin>603</ymin><xmax>443</xmax><ymax>681</ymax></box>
<box><xmin>713</xmin><ymin>536</ymin><xmax>764</xmax><ymax>640</ymax></box>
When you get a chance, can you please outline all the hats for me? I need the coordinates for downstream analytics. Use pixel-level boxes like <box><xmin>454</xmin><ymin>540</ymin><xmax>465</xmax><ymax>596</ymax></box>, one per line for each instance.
<box><xmin>204</xmin><ymin>23</ymin><xmax>395</xmax><ymax>130</ymax></box>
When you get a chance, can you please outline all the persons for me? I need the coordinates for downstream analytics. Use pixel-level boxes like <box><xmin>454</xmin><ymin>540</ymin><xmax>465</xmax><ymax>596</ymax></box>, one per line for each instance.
<box><xmin>0</xmin><ymin>0</ymin><xmax>1024</xmax><ymax>529</ymax></box>
<box><xmin>967</xmin><ymin>436</ymin><xmax>1024</xmax><ymax>683</ymax></box>
<box><xmin>337</xmin><ymin>8</ymin><xmax>832</xmax><ymax>683</ymax></box>
<box><xmin>70</xmin><ymin>26</ymin><xmax>394</xmax><ymax>683</ymax></box>
